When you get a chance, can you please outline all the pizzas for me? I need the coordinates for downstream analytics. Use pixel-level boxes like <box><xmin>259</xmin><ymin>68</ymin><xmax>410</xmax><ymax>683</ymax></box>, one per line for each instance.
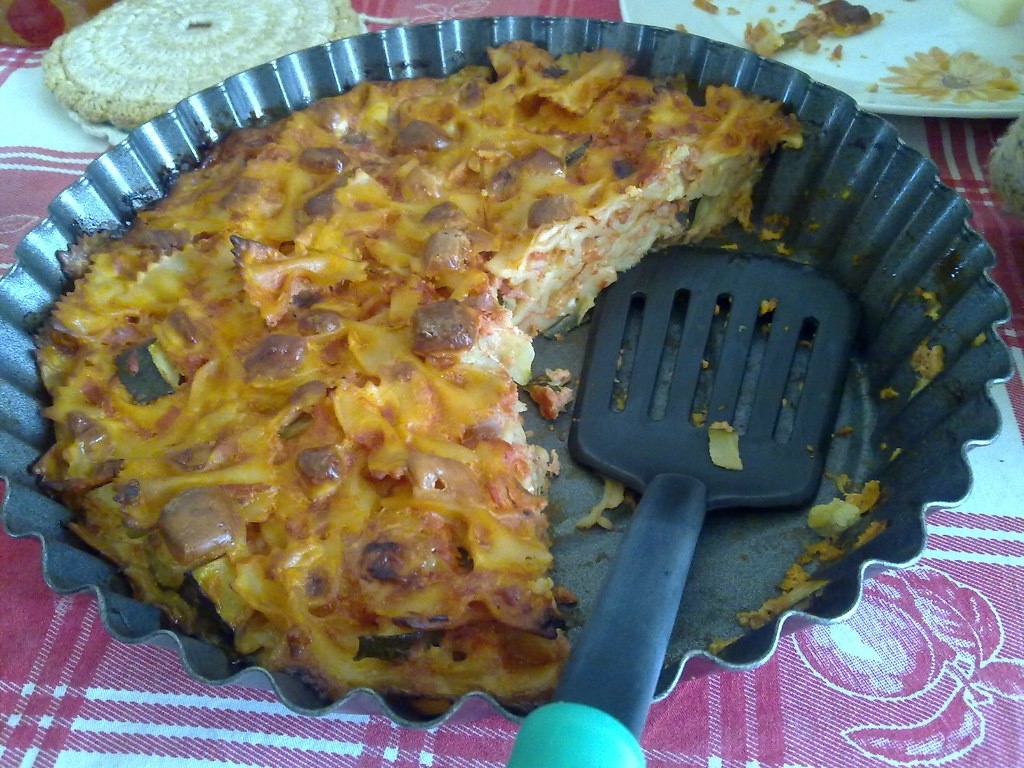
<box><xmin>32</xmin><ymin>37</ymin><xmax>804</xmax><ymax>722</ymax></box>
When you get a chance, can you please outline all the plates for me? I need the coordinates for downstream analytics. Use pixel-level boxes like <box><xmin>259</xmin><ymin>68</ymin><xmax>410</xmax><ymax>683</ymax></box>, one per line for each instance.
<box><xmin>620</xmin><ymin>1</ymin><xmax>1024</xmax><ymax>118</ymax></box>
<box><xmin>0</xmin><ymin>15</ymin><xmax>1015</xmax><ymax>730</ymax></box>
